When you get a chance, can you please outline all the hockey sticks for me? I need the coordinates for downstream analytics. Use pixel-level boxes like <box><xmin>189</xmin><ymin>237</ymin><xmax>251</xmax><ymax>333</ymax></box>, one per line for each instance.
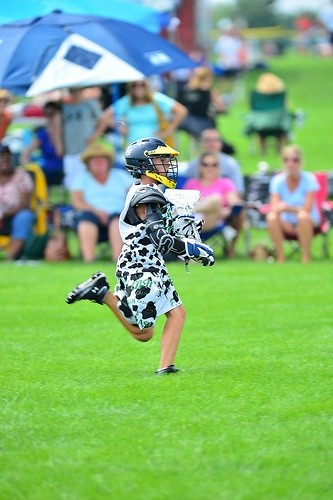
<box><xmin>164</xmin><ymin>188</ymin><xmax>210</xmax><ymax>265</ymax></box>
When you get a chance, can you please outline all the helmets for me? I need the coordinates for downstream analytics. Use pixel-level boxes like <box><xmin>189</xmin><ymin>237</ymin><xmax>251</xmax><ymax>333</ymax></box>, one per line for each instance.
<box><xmin>81</xmin><ymin>140</ymin><xmax>115</xmax><ymax>163</ymax></box>
<box><xmin>125</xmin><ymin>137</ymin><xmax>180</xmax><ymax>189</ymax></box>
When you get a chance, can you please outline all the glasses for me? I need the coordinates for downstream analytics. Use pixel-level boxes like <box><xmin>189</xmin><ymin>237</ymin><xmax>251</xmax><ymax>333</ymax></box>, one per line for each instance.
<box><xmin>201</xmin><ymin>162</ymin><xmax>219</xmax><ymax>167</ymax></box>
<box><xmin>284</xmin><ymin>157</ymin><xmax>298</xmax><ymax>162</ymax></box>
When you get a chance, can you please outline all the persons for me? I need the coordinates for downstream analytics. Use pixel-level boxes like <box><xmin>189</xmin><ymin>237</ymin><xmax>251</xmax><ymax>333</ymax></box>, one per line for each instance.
<box><xmin>266</xmin><ymin>145</ymin><xmax>323</xmax><ymax>261</ymax></box>
<box><xmin>67</xmin><ymin>139</ymin><xmax>139</xmax><ymax>259</ymax></box>
<box><xmin>0</xmin><ymin>145</ymin><xmax>35</xmax><ymax>260</ymax></box>
<box><xmin>181</xmin><ymin>154</ymin><xmax>244</xmax><ymax>256</ymax></box>
<box><xmin>66</xmin><ymin>137</ymin><xmax>218</xmax><ymax>376</ymax></box>
<box><xmin>0</xmin><ymin>16</ymin><xmax>300</xmax><ymax>161</ymax></box>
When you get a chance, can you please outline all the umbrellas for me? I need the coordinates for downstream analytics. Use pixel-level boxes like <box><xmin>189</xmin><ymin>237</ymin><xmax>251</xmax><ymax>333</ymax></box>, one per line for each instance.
<box><xmin>0</xmin><ymin>7</ymin><xmax>202</xmax><ymax>148</ymax></box>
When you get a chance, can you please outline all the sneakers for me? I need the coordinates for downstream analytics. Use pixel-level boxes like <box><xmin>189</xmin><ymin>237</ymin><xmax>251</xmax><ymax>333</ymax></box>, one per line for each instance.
<box><xmin>155</xmin><ymin>365</ymin><xmax>181</xmax><ymax>375</ymax></box>
<box><xmin>66</xmin><ymin>272</ymin><xmax>110</xmax><ymax>304</ymax></box>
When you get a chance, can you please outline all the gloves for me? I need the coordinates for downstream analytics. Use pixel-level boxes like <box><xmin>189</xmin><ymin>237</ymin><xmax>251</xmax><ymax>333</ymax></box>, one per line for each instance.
<box><xmin>179</xmin><ymin>239</ymin><xmax>215</xmax><ymax>267</ymax></box>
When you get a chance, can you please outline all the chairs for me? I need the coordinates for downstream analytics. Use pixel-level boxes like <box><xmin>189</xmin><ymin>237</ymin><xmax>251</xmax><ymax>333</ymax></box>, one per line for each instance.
<box><xmin>242</xmin><ymin>172</ymin><xmax>333</xmax><ymax>260</ymax></box>
